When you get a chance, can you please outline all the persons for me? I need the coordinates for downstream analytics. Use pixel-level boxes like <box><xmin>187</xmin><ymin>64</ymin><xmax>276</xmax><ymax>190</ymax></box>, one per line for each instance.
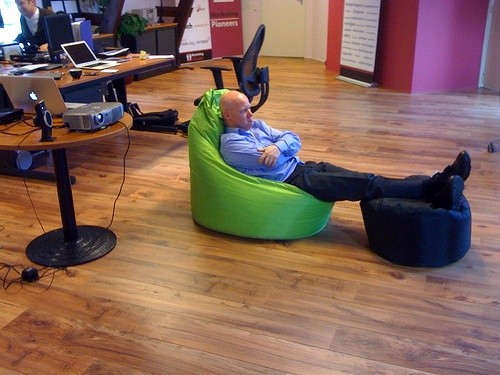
<box><xmin>14</xmin><ymin>0</ymin><xmax>55</xmax><ymax>51</ymax></box>
<box><xmin>220</xmin><ymin>90</ymin><xmax>471</xmax><ymax>211</ymax></box>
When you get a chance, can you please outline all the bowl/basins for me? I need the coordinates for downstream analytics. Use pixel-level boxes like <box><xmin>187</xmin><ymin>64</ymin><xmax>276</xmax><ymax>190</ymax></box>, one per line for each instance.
<box><xmin>70</xmin><ymin>69</ymin><xmax>82</xmax><ymax>79</ymax></box>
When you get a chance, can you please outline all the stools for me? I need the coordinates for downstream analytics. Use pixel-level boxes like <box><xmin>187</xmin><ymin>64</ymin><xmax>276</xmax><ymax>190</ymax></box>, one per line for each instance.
<box><xmin>357</xmin><ymin>174</ymin><xmax>472</xmax><ymax>268</ymax></box>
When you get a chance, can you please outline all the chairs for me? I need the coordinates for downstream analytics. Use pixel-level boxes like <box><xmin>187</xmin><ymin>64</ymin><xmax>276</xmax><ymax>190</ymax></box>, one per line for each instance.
<box><xmin>193</xmin><ymin>24</ymin><xmax>271</xmax><ymax>114</ymax></box>
<box><xmin>187</xmin><ymin>89</ymin><xmax>336</xmax><ymax>240</ymax></box>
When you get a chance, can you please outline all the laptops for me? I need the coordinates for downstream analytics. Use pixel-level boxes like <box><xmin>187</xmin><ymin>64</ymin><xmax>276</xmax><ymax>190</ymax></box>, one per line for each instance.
<box><xmin>61</xmin><ymin>41</ymin><xmax>120</xmax><ymax>70</ymax></box>
<box><xmin>0</xmin><ymin>75</ymin><xmax>85</xmax><ymax>116</ymax></box>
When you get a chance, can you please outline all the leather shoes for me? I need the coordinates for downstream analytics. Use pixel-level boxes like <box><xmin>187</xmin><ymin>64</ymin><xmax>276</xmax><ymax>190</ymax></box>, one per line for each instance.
<box><xmin>443</xmin><ymin>151</ymin><xmax>471</xmax><ymax>181</ymax></box>
<box><xmin>437</xmin><ymin>176</ymin><xmax>464</xmax><ymax>209</ymax></box>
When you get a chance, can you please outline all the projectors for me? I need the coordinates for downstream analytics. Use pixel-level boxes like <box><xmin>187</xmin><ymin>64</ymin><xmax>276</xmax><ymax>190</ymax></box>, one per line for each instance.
<box><xmin>62</xmin><ymin>102</ymin><xmax>123</xmax><ymax>131</ymax></box>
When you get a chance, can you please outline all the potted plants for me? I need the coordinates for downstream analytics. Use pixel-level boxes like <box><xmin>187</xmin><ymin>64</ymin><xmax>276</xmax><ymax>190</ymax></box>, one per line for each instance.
<box><xmin>80</xmin><ymin>0</ymin><xmax>150</xmax><ymax>84</ymax></box>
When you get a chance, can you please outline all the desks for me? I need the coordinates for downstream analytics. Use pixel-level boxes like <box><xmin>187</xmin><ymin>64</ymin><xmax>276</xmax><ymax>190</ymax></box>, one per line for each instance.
<box><xmin>0</xmin><ymin>53</ymin><xmax>176</xmax><ymax>182</ymax></box>
<box><xmin>93</xmin><ymin>32</ymin><xmax>114</xmax><ymax>40</ymax></box>
<box><xmin>0</xmin><ymin>109</ymin><xmax>133</xmax><ymax>267</ymax></box>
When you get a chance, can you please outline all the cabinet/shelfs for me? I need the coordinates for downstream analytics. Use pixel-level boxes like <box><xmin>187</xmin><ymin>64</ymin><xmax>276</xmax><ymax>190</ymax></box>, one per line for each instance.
<box><xmin>121</xmin><ymin>22</ymin><xmax>178</xmax><ymax>80</ymax></box>
